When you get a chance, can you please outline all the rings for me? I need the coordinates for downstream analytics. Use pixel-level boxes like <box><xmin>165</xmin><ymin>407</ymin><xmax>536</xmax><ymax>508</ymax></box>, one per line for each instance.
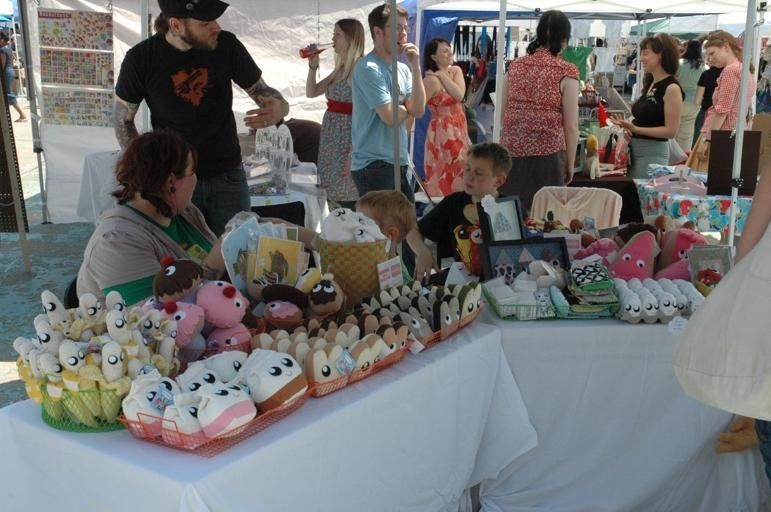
<box><xmin>264</xmin><ymin>121</ymin><xmax>268</xmax><ymax>125</ymax></box>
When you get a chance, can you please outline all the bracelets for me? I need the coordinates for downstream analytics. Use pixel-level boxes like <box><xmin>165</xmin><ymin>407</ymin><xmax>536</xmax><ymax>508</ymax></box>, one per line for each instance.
<box><xmin>309</xmin><ymin>64</ymin><xmax>318</xmax><ymax>70</ymax></box>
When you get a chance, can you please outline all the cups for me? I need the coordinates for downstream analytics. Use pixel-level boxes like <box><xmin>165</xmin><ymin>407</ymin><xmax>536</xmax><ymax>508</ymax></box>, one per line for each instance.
<box><xmin>254</xmin><ymin>124</ymin><xmax>292</xmax><ymax>194</ymax></box>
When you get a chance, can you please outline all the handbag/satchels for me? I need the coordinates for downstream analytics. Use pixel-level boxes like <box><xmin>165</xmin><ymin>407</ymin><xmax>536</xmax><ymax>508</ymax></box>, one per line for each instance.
<box><xmin>672</xmin><ymin>216</ymin><xmax>771</xmax><ymax>422</ymax></box>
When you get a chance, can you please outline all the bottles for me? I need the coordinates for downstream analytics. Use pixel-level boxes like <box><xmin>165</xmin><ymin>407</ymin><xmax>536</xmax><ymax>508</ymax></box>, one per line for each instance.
<box><xmin>300</xmin><ymin>43</ymin><xmax>336</xmax><ymax>59</ymax></box>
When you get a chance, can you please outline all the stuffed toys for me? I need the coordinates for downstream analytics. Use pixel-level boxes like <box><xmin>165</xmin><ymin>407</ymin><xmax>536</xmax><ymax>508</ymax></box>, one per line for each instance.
<box><xmin>12</xmin><ymin>257</ymin><xmax>483</xmax><ymax>449</ymax></box>
<box><xmin>11</xmin><ymin>209</ymin><xmax>724</xmax><ymax>451</ymax></box>
<box><xmin>577</xmin><ymin>221</ymin><xmax>723</xmax><ymax>324</ymax></box>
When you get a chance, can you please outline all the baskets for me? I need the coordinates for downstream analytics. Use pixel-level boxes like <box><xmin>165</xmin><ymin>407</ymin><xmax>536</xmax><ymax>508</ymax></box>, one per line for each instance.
<box><xmin>41</xmin><ymin>291</ymin><xmax>484</xmax><ymax>452</ymax></box>
<box><xmin>41</xmin><ymin>257</ymin><xmax>621</xmax><ymax>458</ymax></box>
<box><xmin>482</xmin><ymin>284</ymin><xmax>620</xmax><ymax>320</ymax></box>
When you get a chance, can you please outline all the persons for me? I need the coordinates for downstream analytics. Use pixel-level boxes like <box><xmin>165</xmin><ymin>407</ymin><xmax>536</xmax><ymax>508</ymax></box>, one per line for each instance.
<box><xmin>609</xmin><ymin>31</ymin><xmax>756</xmax><ymax>179</ymax></box>
<box><xmin>422</xmin><ymin>36</ymin><xmax>471</xmax><ymax>197</ymax></box>
<box><xmin>407</xmin><ymin>142</ymin><xmax>513</xmax><ymax>285</ymax></box>
<box><xmin>76</xmin><ymin>132</ymin><xmax>226</xmax><ymax>308</ymax></box>
<box><xmin>0</xmin><ymin>33</ymin><xmax>27</xmax><ymax>122</ymax></box>
<box><xmin>112</xmin><ymin>0</ymin><xmax>289</xmax><ymax>239</ymax></box>
<box><xmin>717</xmin><ymin>161</ymin><xmax>771</xmax><ymax>483</ymax></box>
<box><xmin>349</xmin><ymin>3</ymin><xmax>426</xmax><ymax>205</ymax></box>
<box><xmin>498</xmin><ymin>10</ymin><xmax>580</xmax><ymax>208</ymax></box>
<box><xmin>469</xmin><ymin>35</ymin><xmax>494</xmax><ymax>81</ymax></box>
<box><xmin>306</xmin><ymin>17</ymin><xmax>365</xmax><ymax>213</ymax></box>
<box><xmin>357</xmin><ymin>190</ymin><xmax>417</xmax><ymax>282</ymax></box>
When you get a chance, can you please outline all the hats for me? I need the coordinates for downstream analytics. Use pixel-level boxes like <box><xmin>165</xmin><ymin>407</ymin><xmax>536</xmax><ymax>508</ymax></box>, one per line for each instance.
<box><xmin>157</xmin><ymin>1</ymin><xmax>230</xmax><ymax>21</ymax></box>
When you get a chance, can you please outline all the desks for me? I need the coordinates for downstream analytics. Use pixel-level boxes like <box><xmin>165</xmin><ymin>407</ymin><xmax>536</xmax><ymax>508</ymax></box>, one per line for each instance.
<box><xmin>476</xmin><ymin>281</ymin><xmax>761</xmax><ymax>512</ymax></box>
<box><xmin>75</xmin><ymin>133</ymin><xmax>759</xmax><ymax>238</ymax></box>
<box><xmin>2</xmin><ymin>320</ymin><xmax>503</xmax><ymax>511</ymax></box>
